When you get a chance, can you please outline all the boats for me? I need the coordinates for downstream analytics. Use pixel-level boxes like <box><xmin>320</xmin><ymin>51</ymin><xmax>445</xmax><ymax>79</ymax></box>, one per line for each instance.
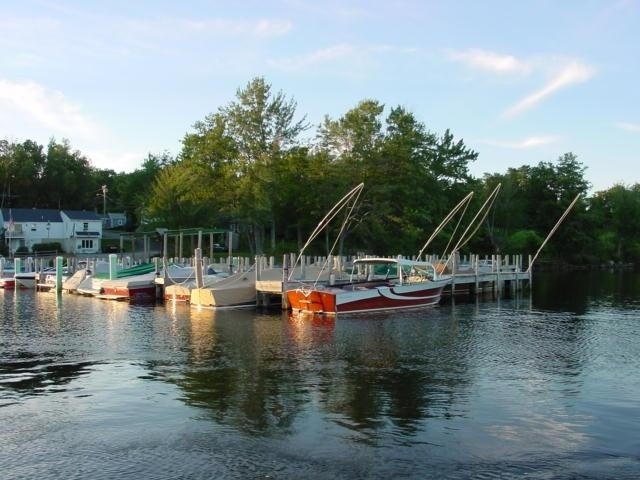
<box><xmin>286</xmin><ymin>257</ymin><xmax>453</xmax><ymax>316</ymax></box>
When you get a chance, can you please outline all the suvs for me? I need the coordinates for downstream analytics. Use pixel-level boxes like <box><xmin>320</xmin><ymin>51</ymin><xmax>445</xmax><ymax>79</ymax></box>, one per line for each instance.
<box><xmin>104</xmin><ymin>243</ymin><xmax>125</xmax><ymax>253</ymax></box>
